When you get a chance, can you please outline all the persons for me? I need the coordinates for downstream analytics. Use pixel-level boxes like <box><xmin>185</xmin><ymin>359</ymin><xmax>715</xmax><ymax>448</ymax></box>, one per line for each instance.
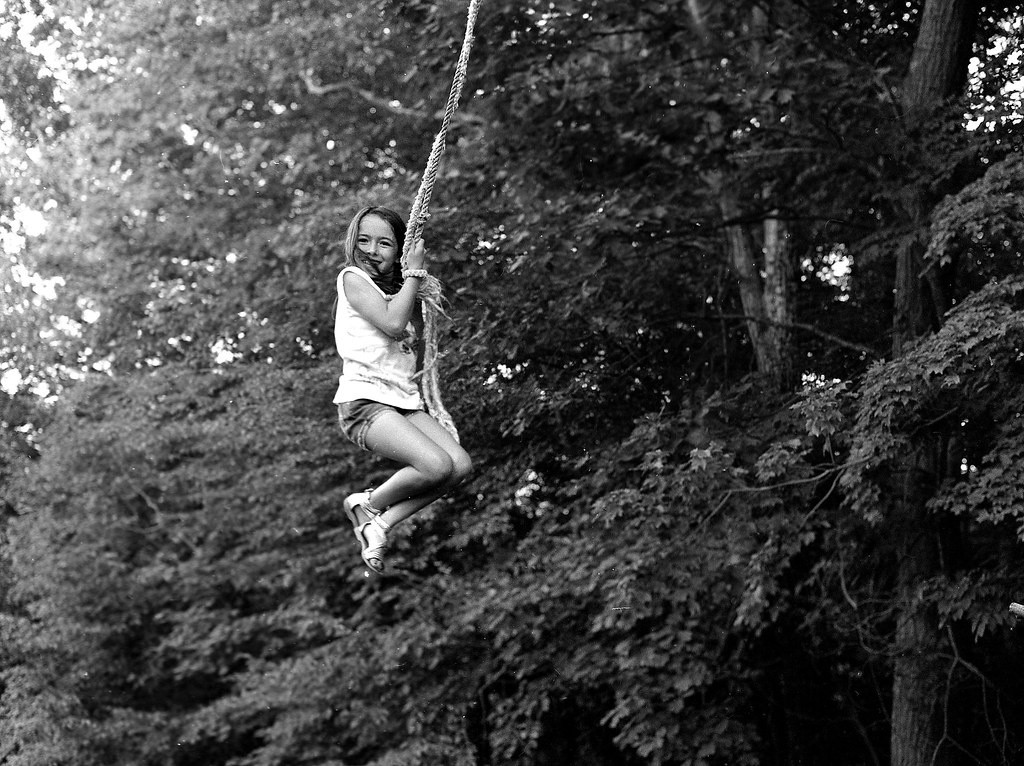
<box><xmin>333</xmin><ymin>204</ymin><xmax>476</xmax><ymax>574</ymax></box>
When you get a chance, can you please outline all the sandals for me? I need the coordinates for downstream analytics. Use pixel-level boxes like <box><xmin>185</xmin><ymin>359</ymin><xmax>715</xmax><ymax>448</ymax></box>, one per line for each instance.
<box><xmin>354</xmin><ymin>513</ymin><xmax>392</xmax><ymax>575</ymax></box>
<box><xmin>344</xmin><ymin>488</ymin><xmax>381</xmax><ymax>529</ymax></box>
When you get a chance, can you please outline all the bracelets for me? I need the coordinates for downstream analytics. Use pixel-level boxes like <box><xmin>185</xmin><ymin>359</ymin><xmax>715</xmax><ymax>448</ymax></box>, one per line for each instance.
<box><xmin>402</xmin><ymin>268</ymin><xmax>428</xmax><ymax>278</ymax></box>
